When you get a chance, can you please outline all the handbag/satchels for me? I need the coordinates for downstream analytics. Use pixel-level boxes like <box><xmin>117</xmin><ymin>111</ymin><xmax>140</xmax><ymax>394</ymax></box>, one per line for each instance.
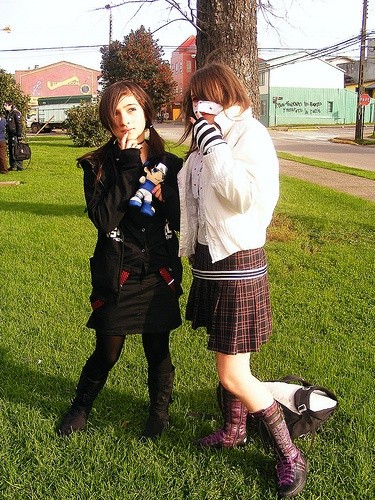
<box><xmin>12</xmin><ymin>137</ymin><xmax>31</xmax><ymax>160</ymax></box>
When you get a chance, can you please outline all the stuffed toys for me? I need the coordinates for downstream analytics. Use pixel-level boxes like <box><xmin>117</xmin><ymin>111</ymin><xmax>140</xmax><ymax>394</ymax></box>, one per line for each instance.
<box><xmin>130</xmin><ymin>163</ymin><xmax>168</xmax><ymax>216</ymax></box>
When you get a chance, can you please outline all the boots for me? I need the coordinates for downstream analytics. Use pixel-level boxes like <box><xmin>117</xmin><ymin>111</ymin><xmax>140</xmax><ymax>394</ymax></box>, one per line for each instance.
<box><xmin>141</xmin><ymin>367</ymin><xmax>176</xmax><ymax>438</ymax></box>
<box><xmin>250</xmin><ymin>399</ymin><xmax>308</xmax><ymax>497</ymax></box>
<box><xmin>197</xmin><ymin>382</ymin><xmax>247</xmax><ymax>447</ymax></box>
<box><xmin>59</xmin><ymin>368</ymin><xmax>107</xmax><ymax>437</ymax></box>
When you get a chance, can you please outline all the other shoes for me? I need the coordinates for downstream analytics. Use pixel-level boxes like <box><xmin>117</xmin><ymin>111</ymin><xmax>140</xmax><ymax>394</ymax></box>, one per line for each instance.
<box><xmin>16</xmin><ymin>167</ymin><xmax>24</xmax><ymax>171</ymax></box>
<box><xmin>7</xmin><ymin>166</ymin><xmax>17</xmax><ymax>170</ymax></box>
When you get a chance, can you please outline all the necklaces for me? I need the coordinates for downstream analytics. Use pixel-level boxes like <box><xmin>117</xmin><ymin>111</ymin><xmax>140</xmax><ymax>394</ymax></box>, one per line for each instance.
<box><xmin>137</xmin><ymin>139</ymin><xmax>145</xmax><ymax>145</ymax></box>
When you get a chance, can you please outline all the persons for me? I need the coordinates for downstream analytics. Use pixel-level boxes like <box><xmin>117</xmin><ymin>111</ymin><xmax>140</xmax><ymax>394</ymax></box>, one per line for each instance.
<box><xmin>171</xmin><ymin>65</ymin><xmax>309</xmax><ymax>497</ymax></box>
<box><xmin>55</xmin><ymin>80</ymin><xmax>184</xmax><ymax>439</ymax></box>
<box><xmin>3</xmin><ymin>100</ymin><xmax>23</xmax><ymax>171</ymax></box>
<box><xmin>0</xmin><ymin>112</ymin><xmax>9</xmax><ymax>174</ymax></box>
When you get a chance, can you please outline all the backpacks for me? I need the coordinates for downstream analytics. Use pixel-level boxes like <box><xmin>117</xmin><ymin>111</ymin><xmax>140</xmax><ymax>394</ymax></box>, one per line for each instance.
<box><xmin>258</xmin><ymin>376</ymin><xmax>339</xmax><ymax>441</ymax></box>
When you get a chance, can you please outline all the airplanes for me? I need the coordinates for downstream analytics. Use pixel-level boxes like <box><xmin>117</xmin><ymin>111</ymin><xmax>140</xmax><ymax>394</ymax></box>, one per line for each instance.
<box><xmin>0</xmin><ymin>25</ymin><xmax>12</xmax><ymax>33</ymax></box>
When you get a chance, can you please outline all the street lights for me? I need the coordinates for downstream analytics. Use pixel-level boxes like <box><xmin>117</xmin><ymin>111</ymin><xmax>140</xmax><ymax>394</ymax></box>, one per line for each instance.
<box><xmin>106</xmin><ymin>4</ymin><xmax>112</xmax><ymax>51</ymax></box>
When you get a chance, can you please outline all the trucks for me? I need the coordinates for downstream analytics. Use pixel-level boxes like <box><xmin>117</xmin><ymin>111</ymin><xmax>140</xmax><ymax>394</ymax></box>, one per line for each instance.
<box><xmin>24</xmin><ymin>102</ymin><xmax>87</xmax><ymax>133</ymax></box>
